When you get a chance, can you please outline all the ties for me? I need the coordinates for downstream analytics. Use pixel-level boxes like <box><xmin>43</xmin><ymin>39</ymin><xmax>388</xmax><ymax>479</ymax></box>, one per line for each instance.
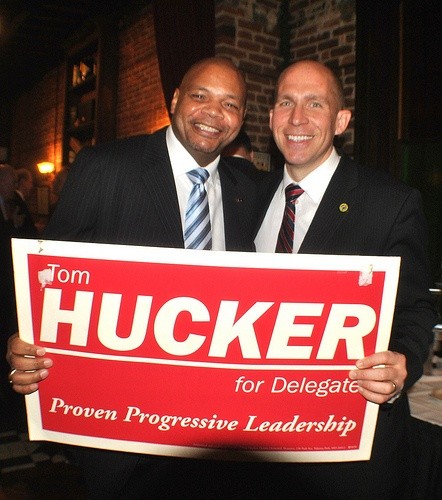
<box><xmin>275</xmin><ymin>184</ymin><xmax>304</xmax><ymax>254</ymax></box>
<box><xmin>184</xmin><ymin>168</ymin><xmax>213</xmax><ymax>251</ymax></box>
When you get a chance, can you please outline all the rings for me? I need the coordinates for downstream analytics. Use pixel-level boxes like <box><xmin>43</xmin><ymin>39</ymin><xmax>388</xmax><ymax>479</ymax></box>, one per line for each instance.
<box><xmin>389</xmin><ymin>380</ymin><xmax>398</xmax><ymax>394</ymax></box>
<box><xmin>8</xmin><ymin>368</ymin><xmax>17</xmax><ymax>383</ymax></box>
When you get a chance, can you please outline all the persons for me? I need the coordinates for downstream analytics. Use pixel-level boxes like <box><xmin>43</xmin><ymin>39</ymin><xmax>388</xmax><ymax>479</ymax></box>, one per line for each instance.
<box><xmin>0</xmin><ymin>164</ymin><xmax>41</xmax><ymax>238</ymax></box>
<box><xmin>47</xmin><ymin>166</ymin><xmax>73</xmax><ymax>221</ymax></box>
<box><xmin>5</xmin><ymin>54</ymin><xmax>258</xmax><ymax>500</ymax></box>
<box><xmin>220</xmin><ymin>132</ymin><xmax>260</xmax><ymax>196</ymax></box>
<box><xmin>233</xmin><ymin>59</ymin><xmax>435</xmax><ymax>500</ymax></box>
